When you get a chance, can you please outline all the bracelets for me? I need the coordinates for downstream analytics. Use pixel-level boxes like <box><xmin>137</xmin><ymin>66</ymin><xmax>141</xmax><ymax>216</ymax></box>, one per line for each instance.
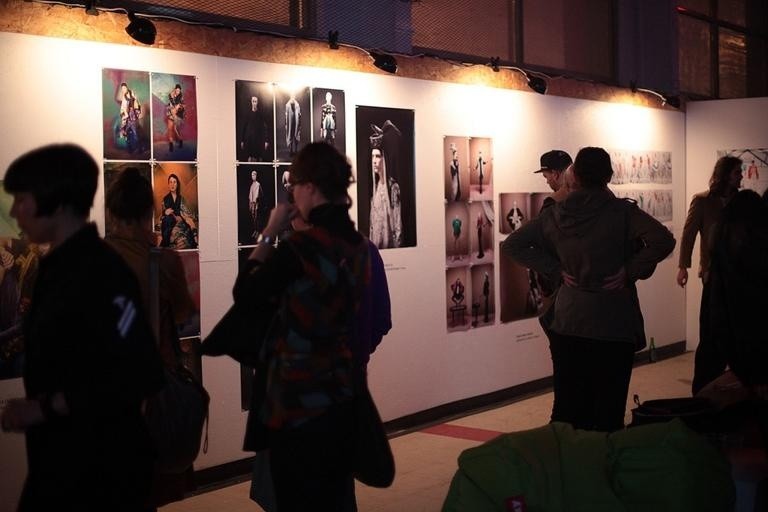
<box><xmin>40</xmin><ymin>393</ymin><xmax>65</xmax><ymax>424</ymax></box>
<box><xmin>256</xmin><ymin>233</ymin><xmax>273</xmax><ymax>245</ymax></box>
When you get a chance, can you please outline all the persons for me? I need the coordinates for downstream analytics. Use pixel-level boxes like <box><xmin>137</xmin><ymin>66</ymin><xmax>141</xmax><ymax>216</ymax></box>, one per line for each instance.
<box><xmin>366</xmin><ymin>146</ymin><xmax>408</xmax><ymax>248</ymax></box>
<box><xmin>245</xmin><ymin>169</ymin><xmax>265</xmax><ymax>239</ymax></box>
<box><xmin>105</xmin><ymin>162</ymin><xmax>203</xmax><ymax>368</ymax></box>
<box><xmin>687</xmin><ymin>186</ymin><xmax>767</xmax><ymax>393</ymax></box>
<box><xmin>229</xmin><ymin>140</ymin><xmax>395</xmax><ymax>512</ymax></box>
<box><xmin>449</xmin><ymin>277</ymin><xmax>466</xmax><ymax>307</ymax></box>
<box><xmin>449</xmin><ymin>214</ymin><xmax>465</xmax><ymax>263</ymax></box>
<box><xmin>240</xmin><ymin>94</ymin><xmax>271</xmax><ymax>161</ymax></box>
<box><xmin>281</xmin><ymin>91</ymin><xmax>303</xmax><ymax>156</ymax></box>
<box><xmin>279</xmin><ymin>169</ymin><xmax>293</xmax><ymax>192</ymax></box>
<box><xmin>116</xmin><ymin>78</ymin><xmax>149</xmax><ymax>152</ymax></box>
<box><xmin>500</xmin><ymin>147</ymin><xmax>677</xmax><ymax>430</ymax></box>
<box><xmin>449</xmin><ymin>149</ymin><xmax>462</xmax><ymax>202</ymax></box>
<box><xmin>475</xmin><ymin>211</ymin><xmax>486</xmax><ymax>259</ymax></box>
<box><xmin>472</xmin><ymin>150</ymin><xmax>495</xmax><ymax>193</ymax></box>
<box><xmin>162</xmin><ymin>83</ymin><xmax>188</xmax><ymax>153</ymax></box>
<box><xmin>680</xmin><ymin>154</ymin><xmax>744</xmax><ymax>408</ymax></box>
<box><xmin>506</xmin><ymin>200</ymin><xmax>524</xmax><ymax>232</ymax></box>
<box><xmin>319</xmin><ymin>90</ymin><xmax>338</xmax><ymax>148</ymax></box>
<box><xmin>157</xmin><ymin>172</ymin><xmax>198</xmax><ymax>249</ymax></box>
<box><xmin>0</xmin><ymin>141</ymin><xmax>150</xmax><ymax>511</ymax></box>
<box><xmin>480</xmin><ymin>272</ymin><xmax>490</xmax><ymax>323</ymax></box>
<box><xmin>748</xmin><ymin>160</ymin><xmax>760</xmax><ymax>180</ymax></box>
<box><xmin>528</xmin><ymin>148</ymin><xmax>573</xmax><ymax>211</ymax></box>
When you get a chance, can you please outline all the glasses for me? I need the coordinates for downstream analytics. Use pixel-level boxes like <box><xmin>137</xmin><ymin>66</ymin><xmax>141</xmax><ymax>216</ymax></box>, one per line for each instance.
<box><xmin>283</xmin><ymin>179</ymin><xmax>303</xmax><ymax>193</ymax></box>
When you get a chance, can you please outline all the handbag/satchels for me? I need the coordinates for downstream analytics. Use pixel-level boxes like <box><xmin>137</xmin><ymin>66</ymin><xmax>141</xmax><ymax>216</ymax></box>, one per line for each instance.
<box><xmin>339</xmin><ymin>374</ymin><xmax>394</xmax><ymax>488</ymax></box>
<box><xmin>159</xmin><ymin>357</ymin><xmax>208</xmax><ymax>477</ymax></box>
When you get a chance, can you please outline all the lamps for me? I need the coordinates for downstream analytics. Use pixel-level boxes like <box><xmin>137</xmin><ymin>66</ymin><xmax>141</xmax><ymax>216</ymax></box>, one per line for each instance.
<box><xmin>85</xmin><ymin>1</ymin><xmax>158</xmax><ymax>45</ymax></box>
<box><xmin>486</xmin><ymin>55</ymin><xmax>547</xmax><ymax>95</ymax></box>
<box><xmin>629</xmin><ymin>79</ymin><xmax>681</xmax><ymax>109</ymax></box>
<box><xmin>327</xmin><ymin>27</ymin><xmax>399</xmax><ymax>74</ymax></box>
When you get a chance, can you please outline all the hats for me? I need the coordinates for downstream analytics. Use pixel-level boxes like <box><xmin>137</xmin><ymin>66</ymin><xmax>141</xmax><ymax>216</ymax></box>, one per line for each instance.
<box><xmin>533</xmin><ymin>151</ymin><xmax>572</xmax><ymax>173</ymax></box>
